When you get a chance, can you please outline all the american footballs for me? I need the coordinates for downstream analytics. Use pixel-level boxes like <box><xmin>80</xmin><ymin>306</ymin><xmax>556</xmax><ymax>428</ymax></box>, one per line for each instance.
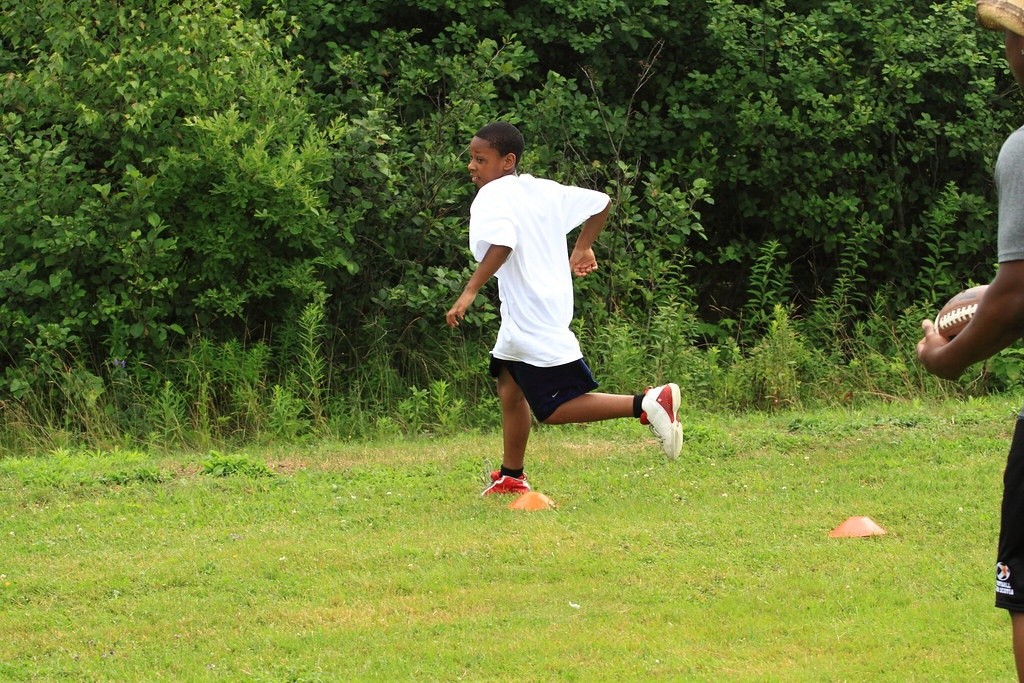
<box><xmin>934</xmin><ymin>284</ymin><xmax>990</xmax><ymax>344</ymax></box>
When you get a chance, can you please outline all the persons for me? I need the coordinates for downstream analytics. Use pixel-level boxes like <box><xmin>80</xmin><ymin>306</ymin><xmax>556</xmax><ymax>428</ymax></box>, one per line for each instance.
<box><xmin>913</xmin><ymin>0</ymin><xmax>1023</xmax><ymax>682</ymax></box>
<box><xmin>445</xmin><ymin>121</ymin><xmax>684</xmax><ymax>499</ymax></box>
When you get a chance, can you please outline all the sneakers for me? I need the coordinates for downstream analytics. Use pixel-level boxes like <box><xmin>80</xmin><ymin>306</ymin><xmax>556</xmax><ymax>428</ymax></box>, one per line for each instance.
<box><xmin>640</xmin><ymin>382</ymin><xmax>684</xmax><ymax>460</ymax></box>
<box><xmin>478</xmin><ymin>470</ymin><xmax>531</xmax><ymax>496</ymax></box>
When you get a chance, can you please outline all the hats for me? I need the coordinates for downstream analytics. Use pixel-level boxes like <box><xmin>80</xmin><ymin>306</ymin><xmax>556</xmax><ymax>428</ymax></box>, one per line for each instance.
<box><xmin>978</xmin><ymin>0</ymin><xmax>1024</xmax><ymax>37</ymax></box>
